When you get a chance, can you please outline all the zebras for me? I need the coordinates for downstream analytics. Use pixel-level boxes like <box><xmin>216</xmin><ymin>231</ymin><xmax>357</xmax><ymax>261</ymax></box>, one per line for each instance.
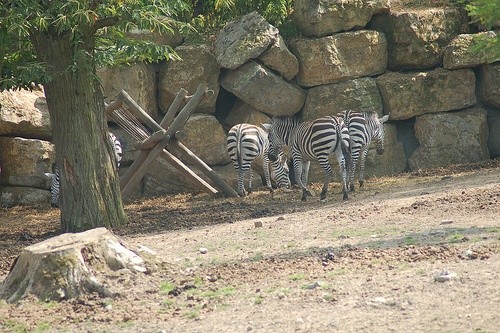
<box><xmin>42</xmin><ymin>131</ymin><xmax>122</xmax><ymax>207</ymax></box>
<box><xmin>260</xmin><ymin>109</ymin><xmax>390</xmax><ymax>200</ymax></box>
<box><xmin>227</xmin><ymin>123</ymin><xmax>290</xmax><ymax>197</ymax></box>
